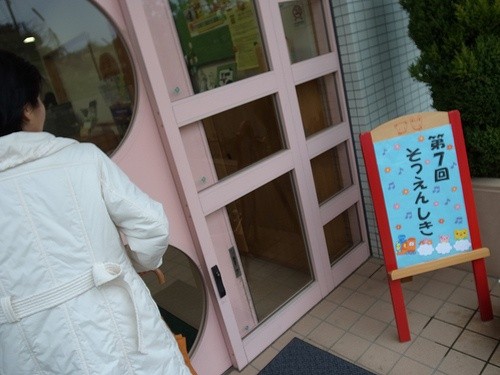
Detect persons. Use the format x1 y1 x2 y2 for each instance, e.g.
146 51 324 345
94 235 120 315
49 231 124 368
1 49 190 374
